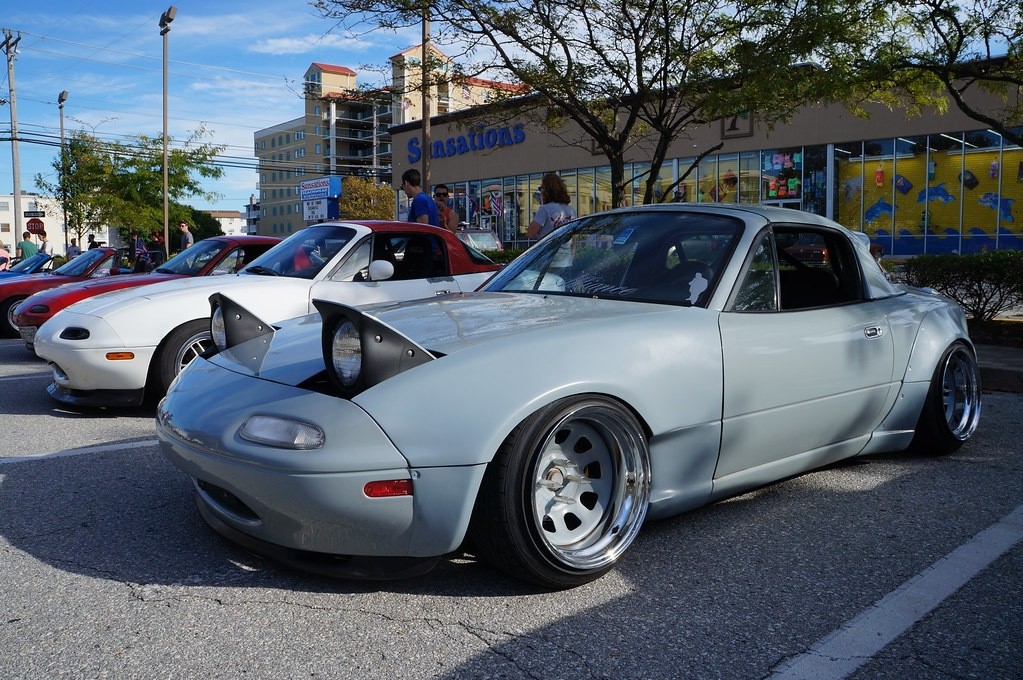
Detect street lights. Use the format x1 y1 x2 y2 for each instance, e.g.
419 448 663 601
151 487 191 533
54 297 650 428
54 90 68 254
157 5 177 258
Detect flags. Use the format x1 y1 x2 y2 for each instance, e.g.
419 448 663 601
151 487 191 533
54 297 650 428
491 193 502 217
469 196 478 218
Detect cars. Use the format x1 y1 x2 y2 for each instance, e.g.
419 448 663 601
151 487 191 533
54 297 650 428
391 218 505 265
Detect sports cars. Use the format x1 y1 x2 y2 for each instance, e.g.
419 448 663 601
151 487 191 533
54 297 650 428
13 234 308 352
33 217 568 413
1 244 131 338
157 198 984 590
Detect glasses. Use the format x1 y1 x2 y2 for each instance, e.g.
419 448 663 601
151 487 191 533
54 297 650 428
179 224 186 227
400 181 405 190
435 191 448 197
538 186 544 190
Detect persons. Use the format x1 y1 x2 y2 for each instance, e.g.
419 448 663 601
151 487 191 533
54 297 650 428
177 219 193 252
38 230 53 256
16 232 38 261
66 238 81 262
526 174 575 241
402 169 439 226
88 234 98 250
151 231 167 265
0 240 10 272
433 184 459 230
